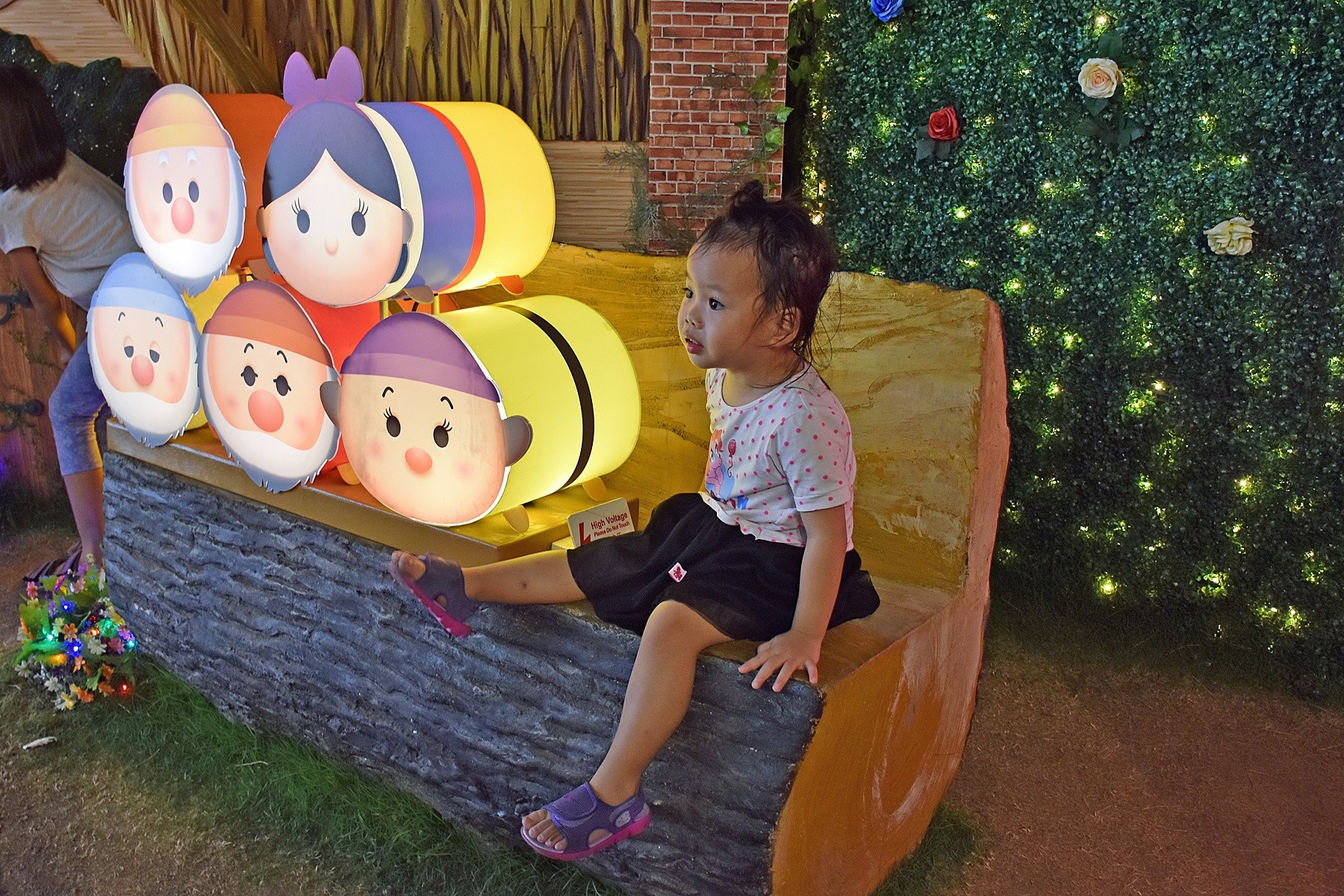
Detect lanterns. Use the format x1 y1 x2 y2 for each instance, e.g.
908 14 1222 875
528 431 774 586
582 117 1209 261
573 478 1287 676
87 46 644 528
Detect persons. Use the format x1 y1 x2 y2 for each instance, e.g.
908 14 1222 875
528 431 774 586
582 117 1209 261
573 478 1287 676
389 180 881 860
0 61 145 598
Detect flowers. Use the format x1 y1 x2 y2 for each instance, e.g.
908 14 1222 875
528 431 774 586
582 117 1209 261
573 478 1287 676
915 103 967 167
1072 30 1145 143
1203 213 1255 259
12 553 138 713
868 0 903 23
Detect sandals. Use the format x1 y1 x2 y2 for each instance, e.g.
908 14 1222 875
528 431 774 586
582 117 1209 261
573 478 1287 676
388 552 483 639
522 783 651 861
24 543 108 600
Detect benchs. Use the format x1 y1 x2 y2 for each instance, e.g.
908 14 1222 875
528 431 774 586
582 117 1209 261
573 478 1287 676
101 236 1012 896
